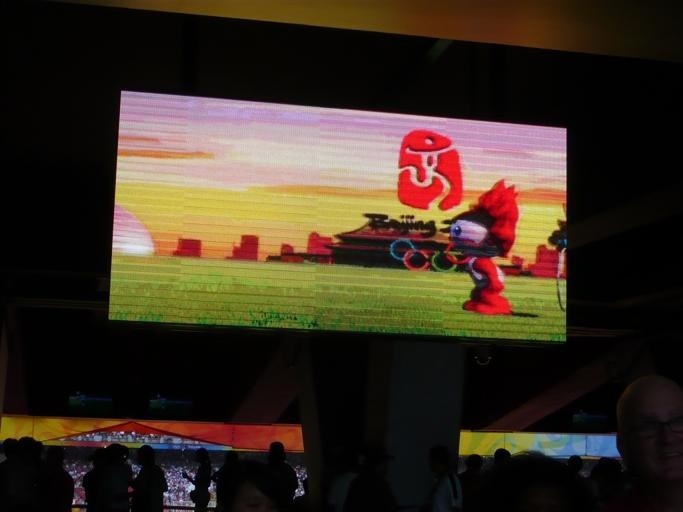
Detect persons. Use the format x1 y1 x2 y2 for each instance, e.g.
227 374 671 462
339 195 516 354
1 425 683 511
589 371 682 511
